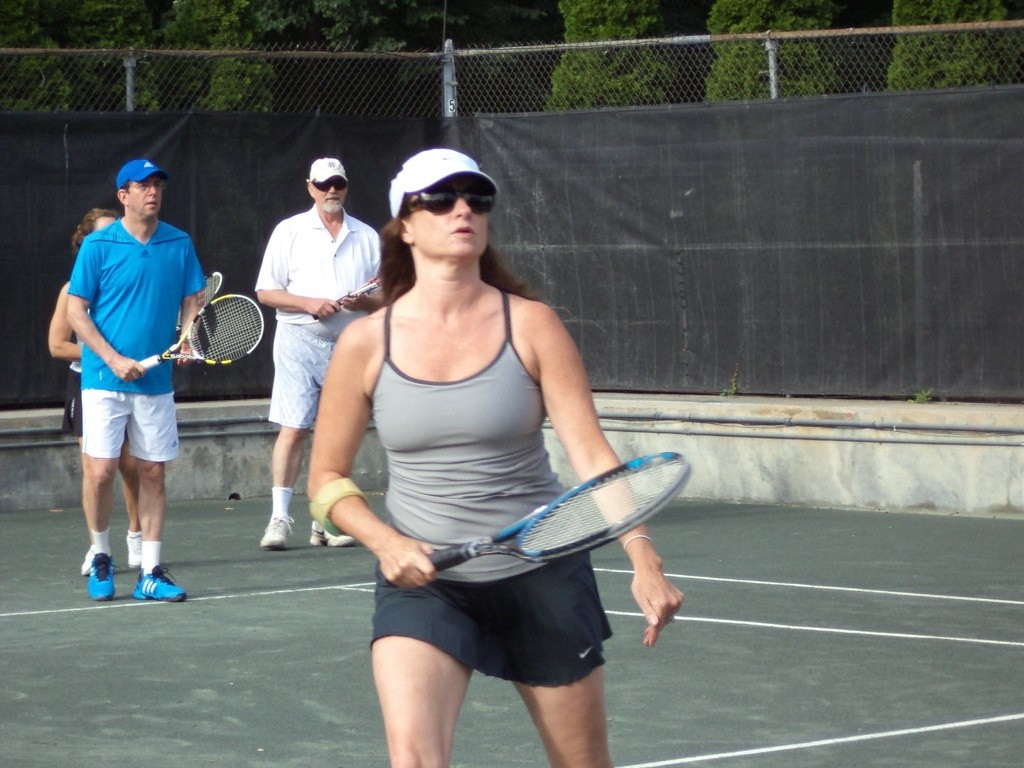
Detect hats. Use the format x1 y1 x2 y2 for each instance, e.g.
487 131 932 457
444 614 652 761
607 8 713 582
389 149 499 219
115 159 169 191
306 158 348 183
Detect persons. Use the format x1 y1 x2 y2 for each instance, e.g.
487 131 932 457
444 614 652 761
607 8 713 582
47 208 142 574
65 160 207 604
307 149 686 768
254 157 384 547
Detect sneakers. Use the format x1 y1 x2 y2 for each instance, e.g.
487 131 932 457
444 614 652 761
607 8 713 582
87 554 114 601
260 516 294 546
81 545 100 575
126 535 142 568
134 566 186 601
310 521 355 547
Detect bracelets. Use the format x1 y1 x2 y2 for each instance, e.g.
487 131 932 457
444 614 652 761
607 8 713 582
623 535 652 551
308 478 369 535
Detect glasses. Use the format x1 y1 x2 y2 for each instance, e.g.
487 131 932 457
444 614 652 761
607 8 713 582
312 180 347 192
404 179 496 213
129 180 167 190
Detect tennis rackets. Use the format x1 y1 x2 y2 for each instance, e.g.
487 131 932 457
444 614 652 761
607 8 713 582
176 270 224 332
386 450 692 589
313 274 385 322
138 293 265 370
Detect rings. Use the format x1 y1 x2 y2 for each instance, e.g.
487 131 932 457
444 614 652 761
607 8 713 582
666 616 675 621
